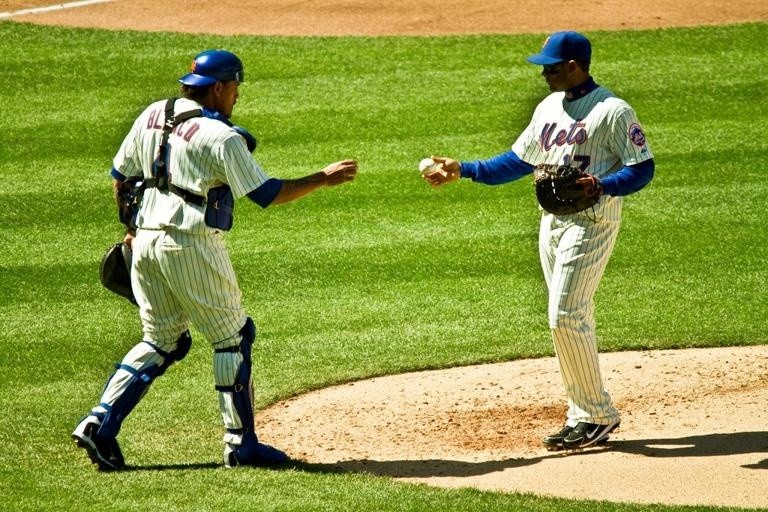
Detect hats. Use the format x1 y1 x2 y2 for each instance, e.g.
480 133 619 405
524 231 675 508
527 31 592 65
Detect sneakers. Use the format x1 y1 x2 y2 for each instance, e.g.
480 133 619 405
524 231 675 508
563 419 624 450
71 416 126 470
224 443 289 467
543 426 574 452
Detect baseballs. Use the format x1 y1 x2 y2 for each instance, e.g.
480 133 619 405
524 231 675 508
419 158 436 175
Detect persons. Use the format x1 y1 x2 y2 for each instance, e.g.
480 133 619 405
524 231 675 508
420 29 658 450
67 50 358 472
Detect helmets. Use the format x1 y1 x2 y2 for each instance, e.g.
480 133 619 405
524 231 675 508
178 49 245 88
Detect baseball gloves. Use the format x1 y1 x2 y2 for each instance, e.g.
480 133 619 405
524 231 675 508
533 164 603 225
100 243 138 308
